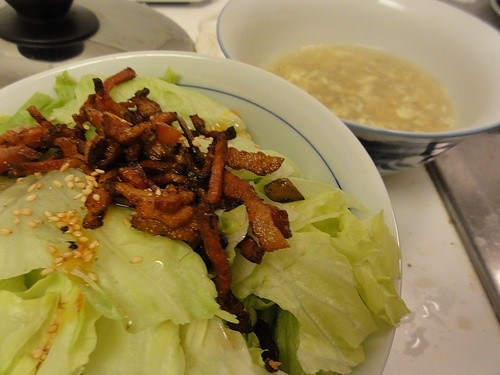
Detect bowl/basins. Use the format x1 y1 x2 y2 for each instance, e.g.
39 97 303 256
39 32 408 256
0 50 401 375
216 0 500 175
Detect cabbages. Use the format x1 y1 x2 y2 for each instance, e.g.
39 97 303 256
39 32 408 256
0 68 413 375
